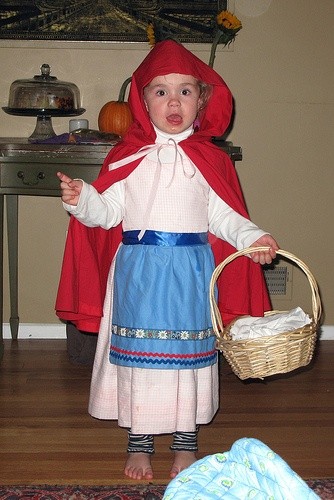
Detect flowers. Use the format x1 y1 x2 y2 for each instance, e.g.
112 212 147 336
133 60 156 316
146 10 243 68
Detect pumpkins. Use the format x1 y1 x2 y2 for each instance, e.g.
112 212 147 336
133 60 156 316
98 77 134 137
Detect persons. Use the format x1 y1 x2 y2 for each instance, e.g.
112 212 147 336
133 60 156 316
55 40 280 480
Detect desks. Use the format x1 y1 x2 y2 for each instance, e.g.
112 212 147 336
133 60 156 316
0 137 242 342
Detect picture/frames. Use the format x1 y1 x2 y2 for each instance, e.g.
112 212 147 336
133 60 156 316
0 0 235 52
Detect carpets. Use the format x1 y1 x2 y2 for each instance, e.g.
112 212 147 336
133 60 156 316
0 476 334 500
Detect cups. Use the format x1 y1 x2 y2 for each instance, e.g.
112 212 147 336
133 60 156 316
69 119 88 132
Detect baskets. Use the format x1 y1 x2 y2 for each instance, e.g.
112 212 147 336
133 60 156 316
208 247 322 381
